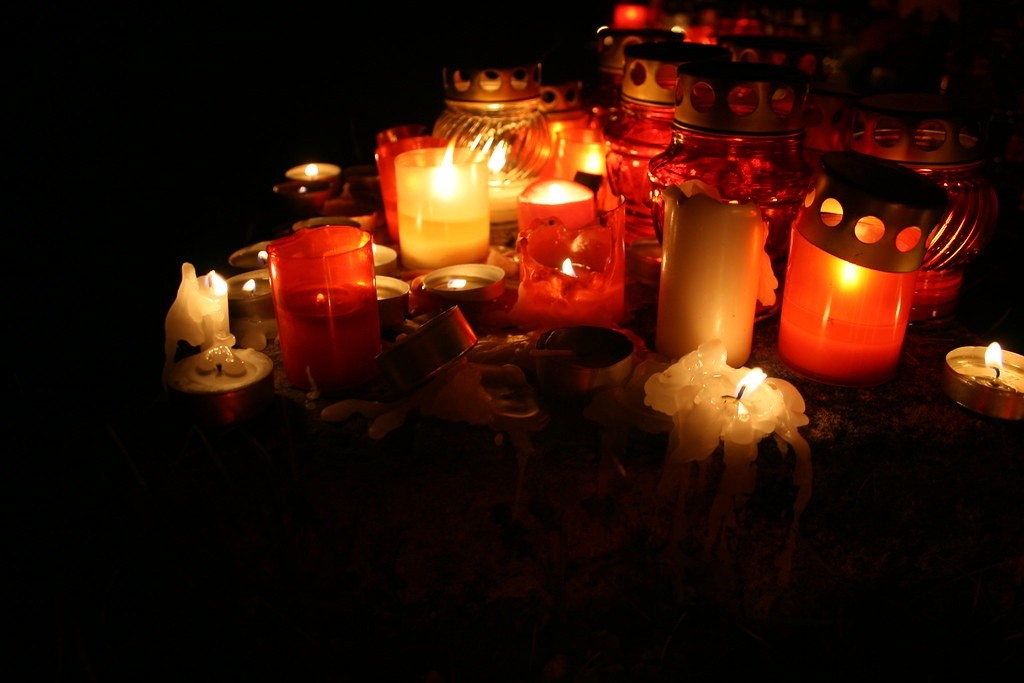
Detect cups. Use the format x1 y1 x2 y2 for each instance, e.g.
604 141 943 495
394 147 490 271
267 225 381 398
654 186 764 367
516 179 626 332
377 135 448 241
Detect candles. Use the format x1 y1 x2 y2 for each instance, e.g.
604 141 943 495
167 139 1024 467
